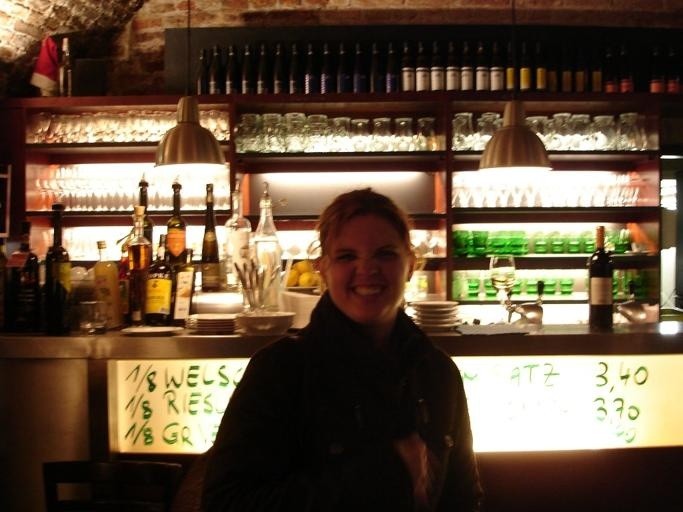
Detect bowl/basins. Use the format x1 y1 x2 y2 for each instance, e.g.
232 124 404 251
240 312 294 334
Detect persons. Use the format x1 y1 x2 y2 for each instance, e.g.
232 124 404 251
194 187 484 511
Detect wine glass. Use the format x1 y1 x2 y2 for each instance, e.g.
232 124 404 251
489 253 514 314
30 110 230 146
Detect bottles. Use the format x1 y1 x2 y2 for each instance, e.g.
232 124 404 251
0 180 70 336
58 35 74 95
94 242 124 330
128 182 281 323
191 38 681 93
589 225 613 330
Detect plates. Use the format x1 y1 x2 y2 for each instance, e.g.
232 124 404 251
186 314 242 334
413 298 461 333
285 285 319 292
120 327 184 335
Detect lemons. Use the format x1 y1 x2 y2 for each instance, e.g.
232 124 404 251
287 260 325 287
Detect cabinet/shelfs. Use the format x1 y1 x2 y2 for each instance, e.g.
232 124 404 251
7 92 659 322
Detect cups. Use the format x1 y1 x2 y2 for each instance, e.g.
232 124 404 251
237 109 439 152
449 111 645 151
76 301 104 331
455 185 645 299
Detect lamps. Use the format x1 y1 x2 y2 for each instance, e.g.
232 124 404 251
153 3 227 181
477 3 555 182
